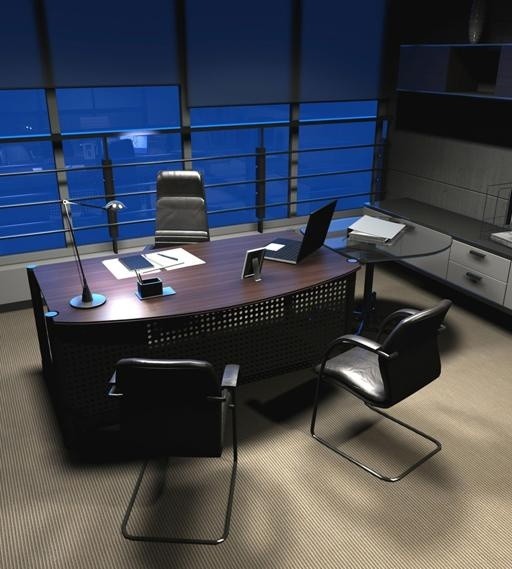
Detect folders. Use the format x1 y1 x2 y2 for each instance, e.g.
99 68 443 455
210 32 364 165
347 214 406 247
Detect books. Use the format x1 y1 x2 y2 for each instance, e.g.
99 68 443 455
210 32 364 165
490 231 512 248
118 255 155 273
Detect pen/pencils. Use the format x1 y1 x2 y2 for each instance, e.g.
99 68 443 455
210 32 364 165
157 254 178 261
135 269 143 283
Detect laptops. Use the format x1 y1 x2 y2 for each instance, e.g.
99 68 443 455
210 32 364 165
262 199 337 265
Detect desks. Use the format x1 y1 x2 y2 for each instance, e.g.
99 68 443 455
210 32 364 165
301 212 453 331
26 228 360 423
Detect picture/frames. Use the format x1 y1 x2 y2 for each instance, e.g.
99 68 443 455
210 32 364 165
241 247 266 279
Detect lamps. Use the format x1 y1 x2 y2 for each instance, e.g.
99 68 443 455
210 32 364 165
62 197 128 308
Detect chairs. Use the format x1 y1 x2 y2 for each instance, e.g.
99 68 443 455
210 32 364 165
138 171 210 254
107 355 240 546
310 300 451 482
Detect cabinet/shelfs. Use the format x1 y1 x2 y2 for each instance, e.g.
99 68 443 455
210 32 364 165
360 205 512 332
